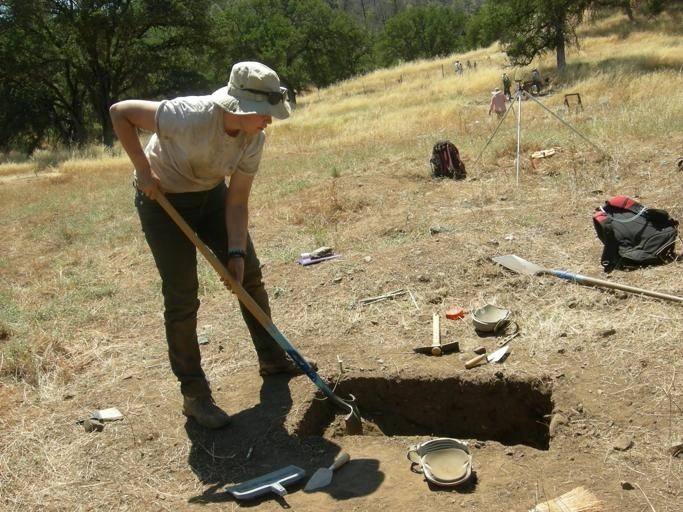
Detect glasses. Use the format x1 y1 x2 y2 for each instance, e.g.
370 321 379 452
239 86 287 103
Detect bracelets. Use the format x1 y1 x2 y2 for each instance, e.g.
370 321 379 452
227 247 247 259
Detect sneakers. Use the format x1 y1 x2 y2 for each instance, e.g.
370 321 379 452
181 394 230 431
258 355 319 379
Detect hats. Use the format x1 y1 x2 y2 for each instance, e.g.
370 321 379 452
489 88 500 96
208 60 294 120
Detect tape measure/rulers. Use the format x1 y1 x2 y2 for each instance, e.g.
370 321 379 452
446 303 463 320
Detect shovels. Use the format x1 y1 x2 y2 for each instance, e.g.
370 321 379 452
226 465 305 500
135 175 362 434
491 254 683 303
303 452 350 490
465 345 509 369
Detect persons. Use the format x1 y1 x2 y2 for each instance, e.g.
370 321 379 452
488 88 508 119
502 73 511 102
454 63 463 78
454 60 459 78
110 59 316 429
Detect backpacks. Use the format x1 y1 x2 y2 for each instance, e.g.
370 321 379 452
430 138 467 181
590 194 678 275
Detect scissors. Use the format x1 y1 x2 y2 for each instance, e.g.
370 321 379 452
362 288 406 306
